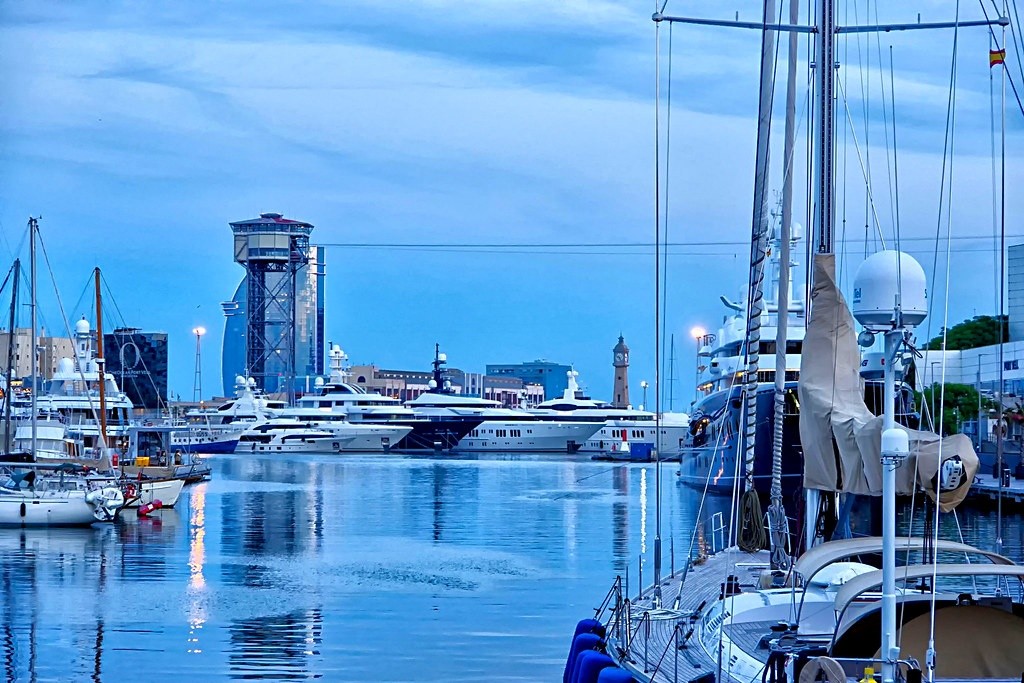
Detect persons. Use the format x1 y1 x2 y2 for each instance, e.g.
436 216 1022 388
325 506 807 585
160 451 166 467
174 449 182 465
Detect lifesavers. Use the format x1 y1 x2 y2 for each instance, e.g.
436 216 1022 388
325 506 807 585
798 655 847 683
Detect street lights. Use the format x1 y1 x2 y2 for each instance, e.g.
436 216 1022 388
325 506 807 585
690 326 707 402
191 327 206 403
641 381 649 410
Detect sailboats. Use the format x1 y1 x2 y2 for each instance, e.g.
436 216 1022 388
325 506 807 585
0 1 1024 680
